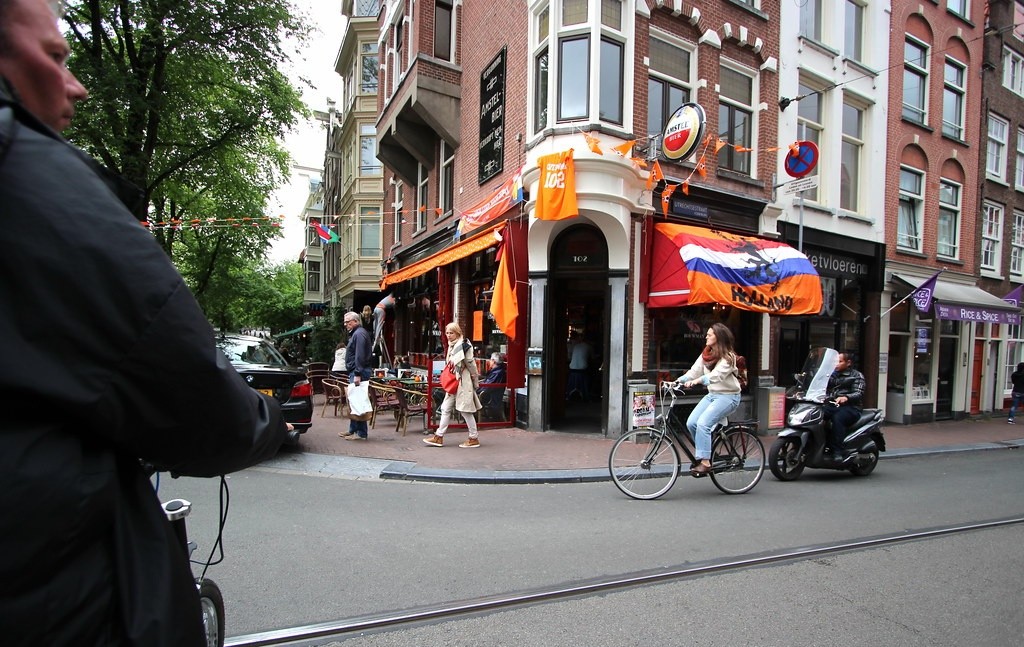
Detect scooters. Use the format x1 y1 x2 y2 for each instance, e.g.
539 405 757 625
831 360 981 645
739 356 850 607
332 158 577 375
768 346 887 482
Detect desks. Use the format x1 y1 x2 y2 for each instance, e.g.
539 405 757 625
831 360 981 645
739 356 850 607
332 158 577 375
401 380 439 422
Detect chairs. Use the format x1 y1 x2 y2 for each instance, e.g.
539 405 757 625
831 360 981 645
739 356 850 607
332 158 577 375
477 383 509 429
306 363 436 438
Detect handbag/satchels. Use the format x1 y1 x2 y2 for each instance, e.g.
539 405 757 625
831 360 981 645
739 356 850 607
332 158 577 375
440 360 458 395
343 380 373 422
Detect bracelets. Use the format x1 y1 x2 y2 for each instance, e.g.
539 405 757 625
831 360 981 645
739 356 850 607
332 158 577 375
843 395 849 402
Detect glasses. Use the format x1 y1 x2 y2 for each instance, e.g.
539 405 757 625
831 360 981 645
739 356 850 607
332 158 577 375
342 319 355 323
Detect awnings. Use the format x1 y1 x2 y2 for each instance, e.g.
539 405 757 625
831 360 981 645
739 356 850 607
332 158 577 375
644 223 824 318
379 218 510 290
891 274 1022 327
273 323 315 341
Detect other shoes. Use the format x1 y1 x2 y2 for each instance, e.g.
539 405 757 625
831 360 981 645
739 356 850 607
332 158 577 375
1007 418 1016 425
690 463 711 474
833 451 844 463
344 432 365 441
338 431 353 437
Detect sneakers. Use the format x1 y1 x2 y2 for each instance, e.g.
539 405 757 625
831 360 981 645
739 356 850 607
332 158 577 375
422 434 444 447
458 437 480 448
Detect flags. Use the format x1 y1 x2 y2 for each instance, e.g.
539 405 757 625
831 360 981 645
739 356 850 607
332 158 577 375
1001 284 1023 308
311 220 342 244
490 223 518 341
910 270 943 313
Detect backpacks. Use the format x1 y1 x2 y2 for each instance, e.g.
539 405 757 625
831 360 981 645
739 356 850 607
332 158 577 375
732 354 748 386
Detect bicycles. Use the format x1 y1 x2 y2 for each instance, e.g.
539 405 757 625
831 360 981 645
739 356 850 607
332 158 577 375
608 380 767 500
128 425 304 647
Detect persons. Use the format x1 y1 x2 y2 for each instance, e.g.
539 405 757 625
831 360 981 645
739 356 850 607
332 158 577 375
361 305 375 338
338 311 373 441
396 355 411 369
664 324 742 474
423 321 486 448
474 353 506 406
1007 362 1024 425
332 343 348 404
558 330 601 407
801 352 866 460
1 1 295 647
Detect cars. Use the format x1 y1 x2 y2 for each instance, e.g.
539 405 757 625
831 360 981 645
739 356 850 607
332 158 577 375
210 330 315 437
